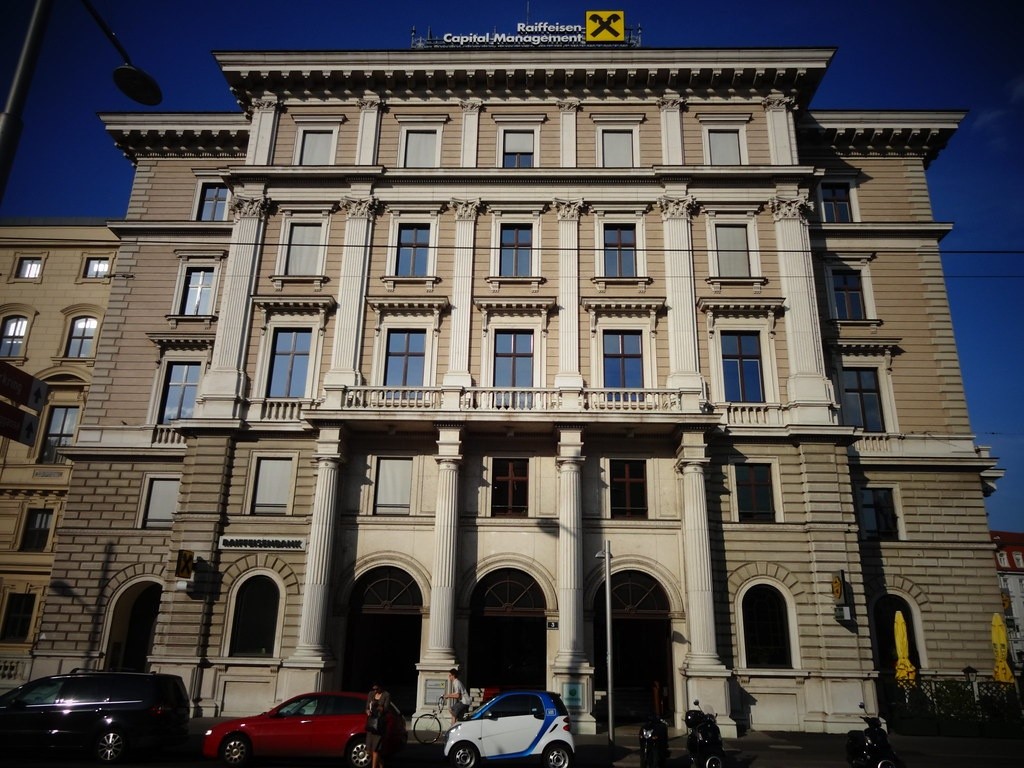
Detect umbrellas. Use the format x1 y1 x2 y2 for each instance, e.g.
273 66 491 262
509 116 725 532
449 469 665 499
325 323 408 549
990 613 1015 722
894 610 916 708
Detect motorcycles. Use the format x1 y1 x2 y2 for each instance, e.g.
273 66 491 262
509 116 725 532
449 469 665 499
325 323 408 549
638 719 669 768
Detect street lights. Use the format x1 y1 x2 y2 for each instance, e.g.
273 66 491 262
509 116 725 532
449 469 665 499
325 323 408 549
1 1 166 209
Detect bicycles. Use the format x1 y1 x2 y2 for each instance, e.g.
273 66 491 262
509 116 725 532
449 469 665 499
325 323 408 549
412 694 471 744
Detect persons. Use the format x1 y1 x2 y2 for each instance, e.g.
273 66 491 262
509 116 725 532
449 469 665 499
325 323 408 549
365 681 391 768
443 668 471 721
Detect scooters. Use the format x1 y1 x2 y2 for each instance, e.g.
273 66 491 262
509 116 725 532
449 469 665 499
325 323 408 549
844 702 899 768
684 700 727 768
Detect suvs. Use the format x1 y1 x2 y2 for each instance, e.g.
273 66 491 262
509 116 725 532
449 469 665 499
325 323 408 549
0 668 191 768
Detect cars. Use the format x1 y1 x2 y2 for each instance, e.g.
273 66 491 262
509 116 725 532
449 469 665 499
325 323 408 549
203 691 407 768
443 691 575 768
